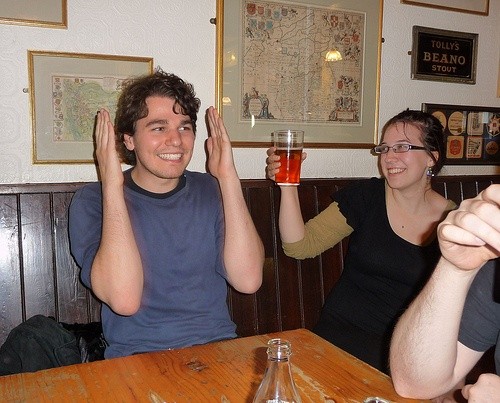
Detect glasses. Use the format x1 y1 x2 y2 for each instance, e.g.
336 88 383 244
374 143 434 154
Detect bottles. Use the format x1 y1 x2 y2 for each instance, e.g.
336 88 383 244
254 338 301 403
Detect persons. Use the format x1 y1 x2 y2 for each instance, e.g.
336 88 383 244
67 69 265 361
267 107 460 374
388 184 500 403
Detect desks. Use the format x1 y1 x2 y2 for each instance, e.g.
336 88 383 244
0 329 430 403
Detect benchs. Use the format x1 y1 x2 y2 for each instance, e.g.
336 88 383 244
0 175 500 384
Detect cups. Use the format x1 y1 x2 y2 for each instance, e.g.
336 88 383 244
273 129 305 186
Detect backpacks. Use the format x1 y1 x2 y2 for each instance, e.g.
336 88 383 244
0 314 88 378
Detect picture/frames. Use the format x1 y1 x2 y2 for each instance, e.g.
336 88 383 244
400 0 490 16
0 0 67 29
411 25 478 84
421 103 500 165
27 49 153 165
216 0 384 148
